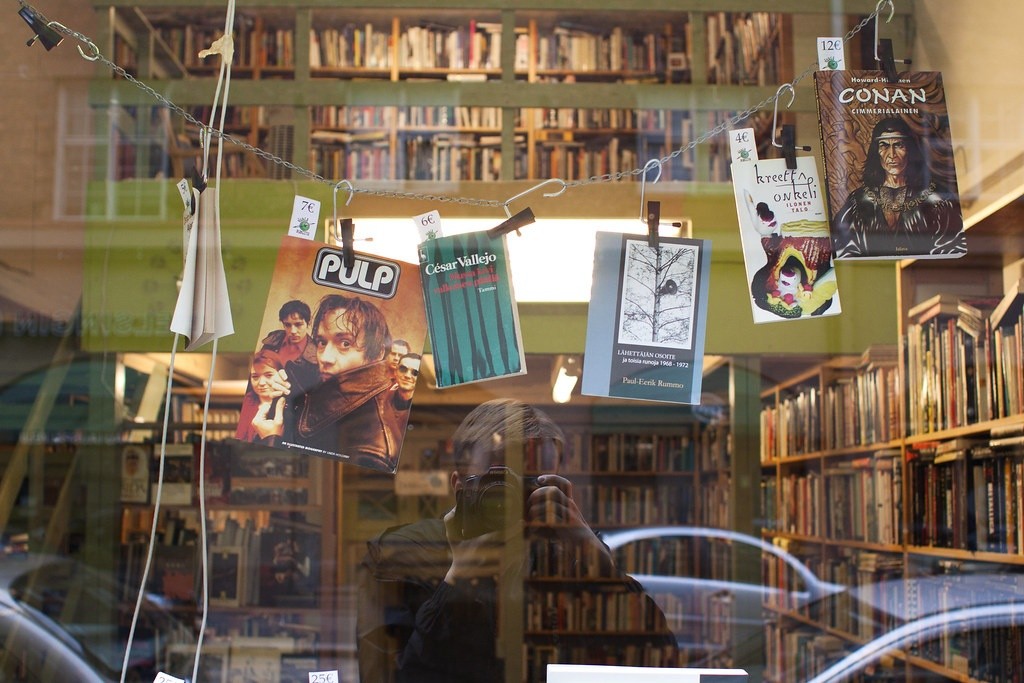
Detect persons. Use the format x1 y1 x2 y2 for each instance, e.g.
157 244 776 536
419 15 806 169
347 396 682 683
235 291 423 473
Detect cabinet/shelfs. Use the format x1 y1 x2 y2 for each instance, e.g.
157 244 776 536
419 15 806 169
86 0 795 188
2 185 1024 677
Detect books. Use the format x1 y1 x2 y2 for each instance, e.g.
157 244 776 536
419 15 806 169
519 420 737 683
167 182 237 353
724 156 843 325
230 234 429 473
580 231 714 407
119 391 322 683
416 229 529 391
109 8 796 188
812 69 969 261
758 287 1024 682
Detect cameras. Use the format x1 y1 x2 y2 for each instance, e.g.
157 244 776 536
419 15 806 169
456 465 559 543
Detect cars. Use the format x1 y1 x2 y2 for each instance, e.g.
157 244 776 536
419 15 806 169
684 569 1024 683
600 530 854 657
0 547 223 683
